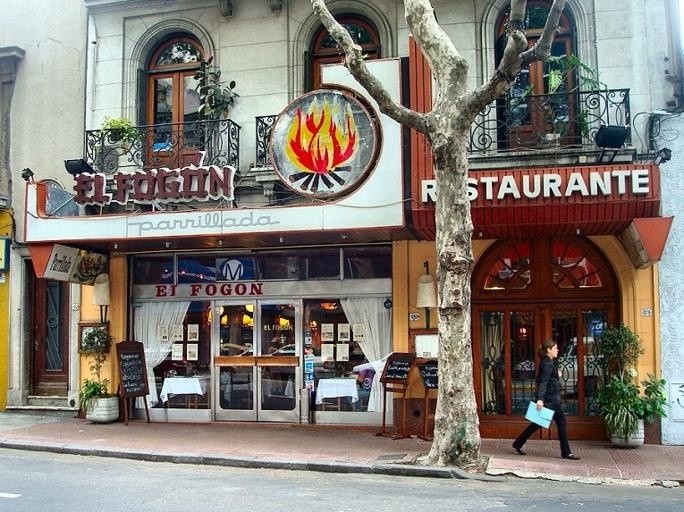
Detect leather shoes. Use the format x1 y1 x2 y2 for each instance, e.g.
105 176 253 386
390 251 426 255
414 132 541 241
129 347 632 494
512 441 528 456
564 452 581 460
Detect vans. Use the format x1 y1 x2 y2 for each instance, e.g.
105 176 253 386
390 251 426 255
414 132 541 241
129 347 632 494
556 336 610 397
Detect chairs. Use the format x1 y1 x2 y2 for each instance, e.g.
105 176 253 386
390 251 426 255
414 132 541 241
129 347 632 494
228 367 252 410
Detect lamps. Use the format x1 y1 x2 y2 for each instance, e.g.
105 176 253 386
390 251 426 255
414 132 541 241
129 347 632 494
63 157 96 178
594 124 629 164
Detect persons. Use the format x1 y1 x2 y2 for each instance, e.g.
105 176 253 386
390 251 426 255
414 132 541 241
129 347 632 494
511 339 580 460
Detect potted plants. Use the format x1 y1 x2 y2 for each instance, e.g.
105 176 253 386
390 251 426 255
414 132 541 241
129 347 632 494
97 113 144 148
78 319 122 425
589 321 671 450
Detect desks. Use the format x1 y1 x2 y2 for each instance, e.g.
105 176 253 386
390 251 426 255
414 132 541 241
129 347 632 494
316 376 359 410
222 372 255 399
163 374 209 409
260 378 297 409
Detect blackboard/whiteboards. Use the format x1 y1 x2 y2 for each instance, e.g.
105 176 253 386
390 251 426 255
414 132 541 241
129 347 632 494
116 341 150 398
380 352 438 389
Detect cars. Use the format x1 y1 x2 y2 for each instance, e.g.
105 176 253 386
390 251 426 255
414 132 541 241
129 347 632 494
219 343 394 392
153 356 192 379
